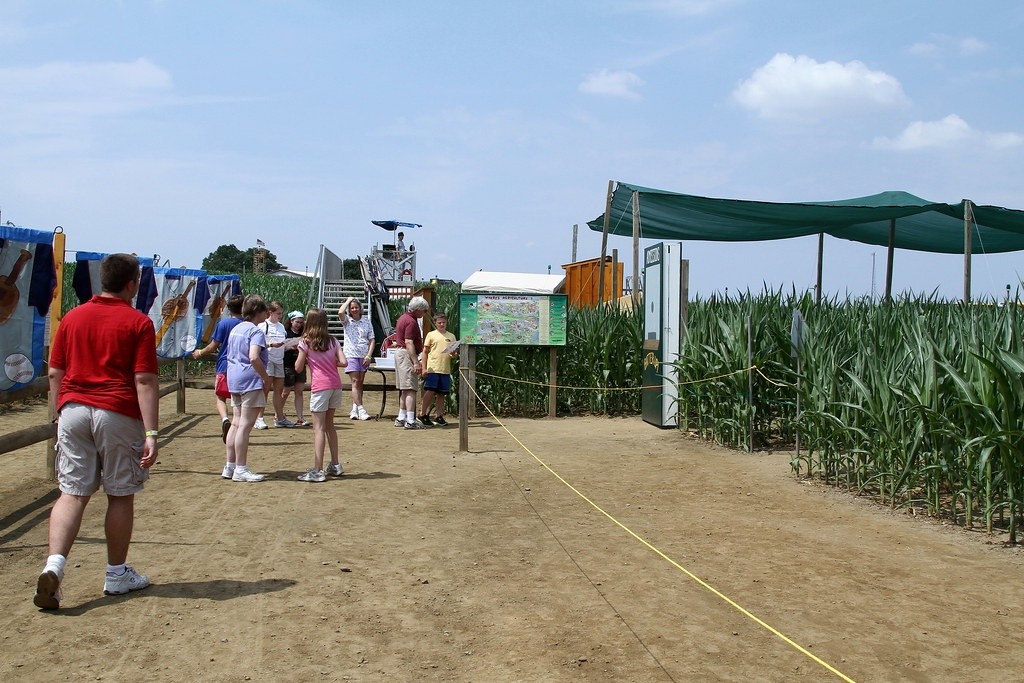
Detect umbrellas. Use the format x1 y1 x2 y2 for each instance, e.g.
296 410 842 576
371 220 422 281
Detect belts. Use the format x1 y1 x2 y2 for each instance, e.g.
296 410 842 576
398 346 406 349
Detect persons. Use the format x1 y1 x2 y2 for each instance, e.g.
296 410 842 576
294 309 348 482
338 296 375 421
273 310 310 426
416 312 458 426
190 294 245 444
254 301 294 430
394 296 430 431
34 252 160 610
221 294 270 483
395 231 407 282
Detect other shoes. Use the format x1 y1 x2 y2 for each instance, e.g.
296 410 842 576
349 409 358 420
325 462 345 476
221 466 235 478
254 417 268 429
275 418 294 427
394 417 406 427
416 413 434 427
232 469 264 482
221 419 231 444
358 409 371 420
298 467 326 481
404 421 426 430
434 415 448 426
296 416 309 426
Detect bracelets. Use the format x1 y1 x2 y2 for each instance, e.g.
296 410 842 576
146 430 159 437
198 350 203 357
269 343 273 347
366 356 371 360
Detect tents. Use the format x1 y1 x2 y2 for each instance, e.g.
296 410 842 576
586 181 1024 321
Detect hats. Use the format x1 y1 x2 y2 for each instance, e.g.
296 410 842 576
288 310 304 320
398 232 404 236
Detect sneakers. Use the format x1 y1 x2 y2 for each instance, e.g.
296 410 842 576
32 563 64 610
103 566 149 594
